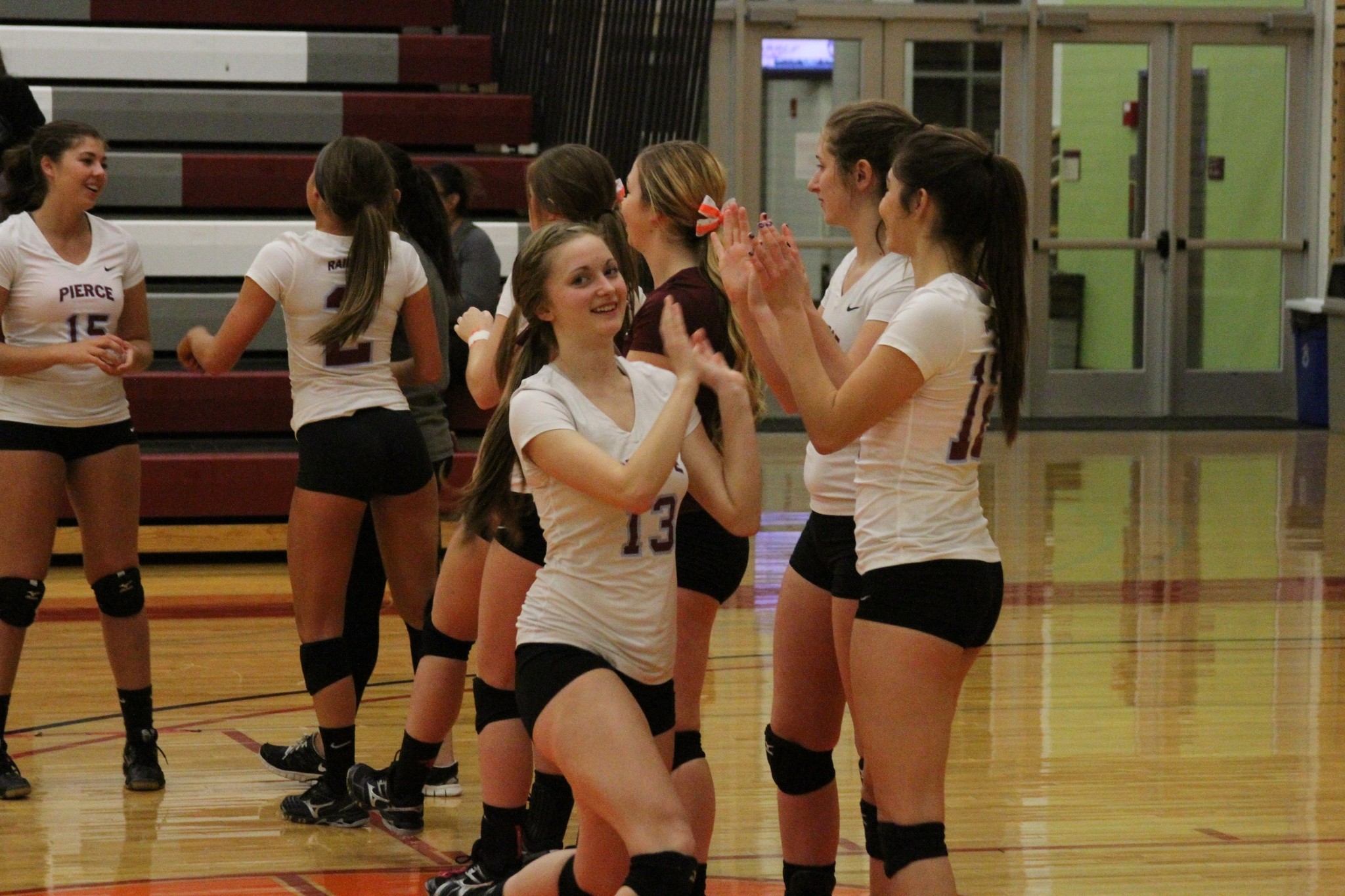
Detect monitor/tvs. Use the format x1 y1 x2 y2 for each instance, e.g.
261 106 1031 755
759 38 835 76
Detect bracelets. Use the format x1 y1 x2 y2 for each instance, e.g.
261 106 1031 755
468 329 491 347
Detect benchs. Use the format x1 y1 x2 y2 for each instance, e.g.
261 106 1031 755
0 1 544 570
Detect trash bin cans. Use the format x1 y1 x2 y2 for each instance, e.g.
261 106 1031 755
1285 300 1328 427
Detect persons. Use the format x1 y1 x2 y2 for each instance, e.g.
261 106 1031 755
0 118 170 798
178 135 647 835
432 224 761 896
747 128 1026 895
619 142 752 896
708 100 985 896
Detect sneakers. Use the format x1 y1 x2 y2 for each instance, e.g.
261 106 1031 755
471 831 563 867
346 750 426 835
426 854 523 896
280 776 372 826
0 741 32 799
121 728 168 790
422 760 463 798
260 732 327 785
